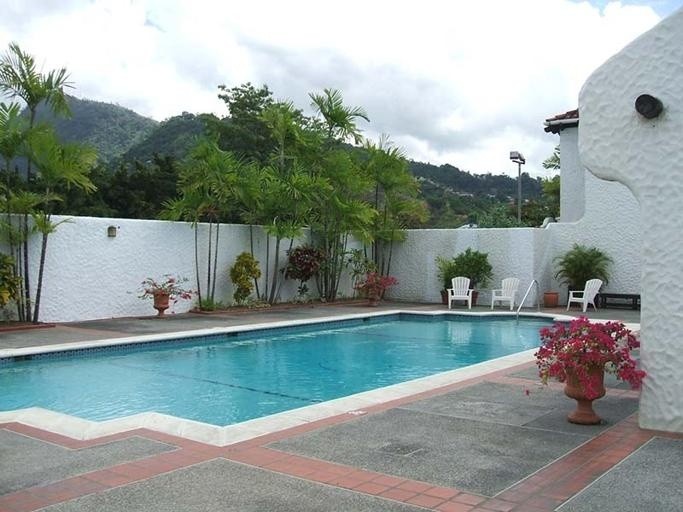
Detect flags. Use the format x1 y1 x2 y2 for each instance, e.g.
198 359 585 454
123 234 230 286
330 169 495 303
562 353 609 424
543 292 558 308
152 292 169 318
367 297 378 306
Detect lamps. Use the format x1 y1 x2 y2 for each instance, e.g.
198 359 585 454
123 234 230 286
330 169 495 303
446 276 474 310
491 277 520 310
566 278 603 313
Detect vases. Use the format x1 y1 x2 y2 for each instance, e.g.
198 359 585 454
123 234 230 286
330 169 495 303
352 270 400 297
125 273 200 307
524 313 648 402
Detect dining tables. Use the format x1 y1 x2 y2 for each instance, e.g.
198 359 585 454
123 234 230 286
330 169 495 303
552 242 614 308
433 247 494 306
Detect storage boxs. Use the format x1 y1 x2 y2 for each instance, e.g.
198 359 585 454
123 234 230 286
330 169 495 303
601 292 640 310
